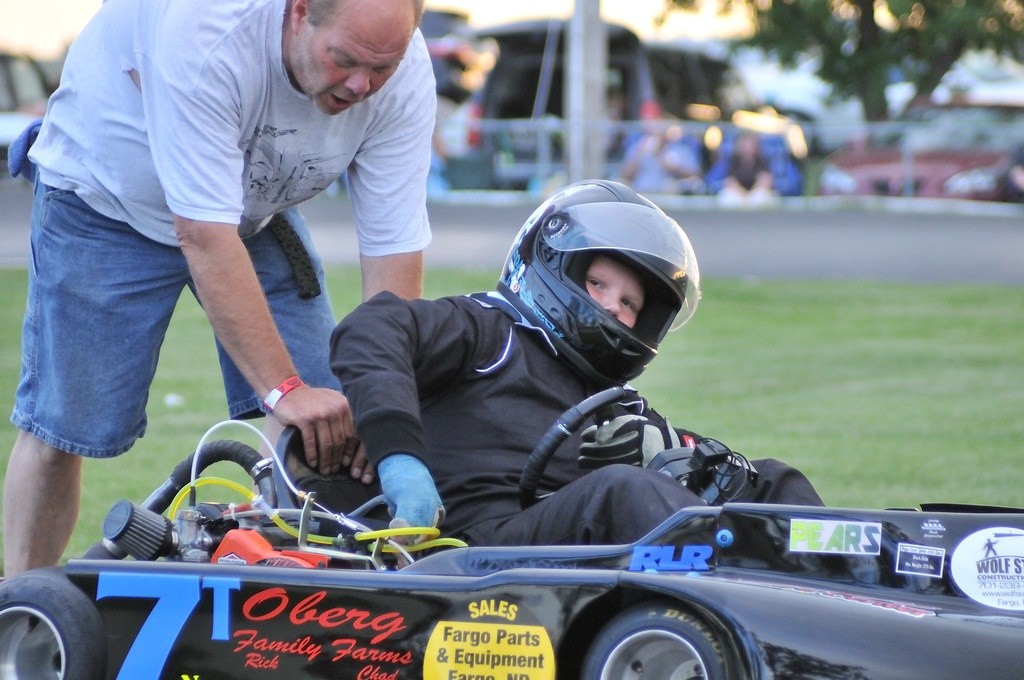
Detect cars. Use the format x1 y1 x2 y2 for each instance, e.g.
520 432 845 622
817 67 1024 205
0 6 841 198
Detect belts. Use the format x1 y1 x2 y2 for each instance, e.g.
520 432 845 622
268 213 321 299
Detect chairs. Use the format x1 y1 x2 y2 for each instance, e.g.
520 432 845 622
276 424 392 536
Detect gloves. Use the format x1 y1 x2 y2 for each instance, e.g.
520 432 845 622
578 383 705 487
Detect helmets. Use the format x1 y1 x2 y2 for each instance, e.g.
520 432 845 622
496 179 702 390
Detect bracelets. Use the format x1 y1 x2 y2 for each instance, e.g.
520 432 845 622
262 376 304 411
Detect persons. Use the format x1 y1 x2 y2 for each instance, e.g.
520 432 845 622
722 130 773 194
622 128 700 189
329 178 825 558
3 0 437 577
